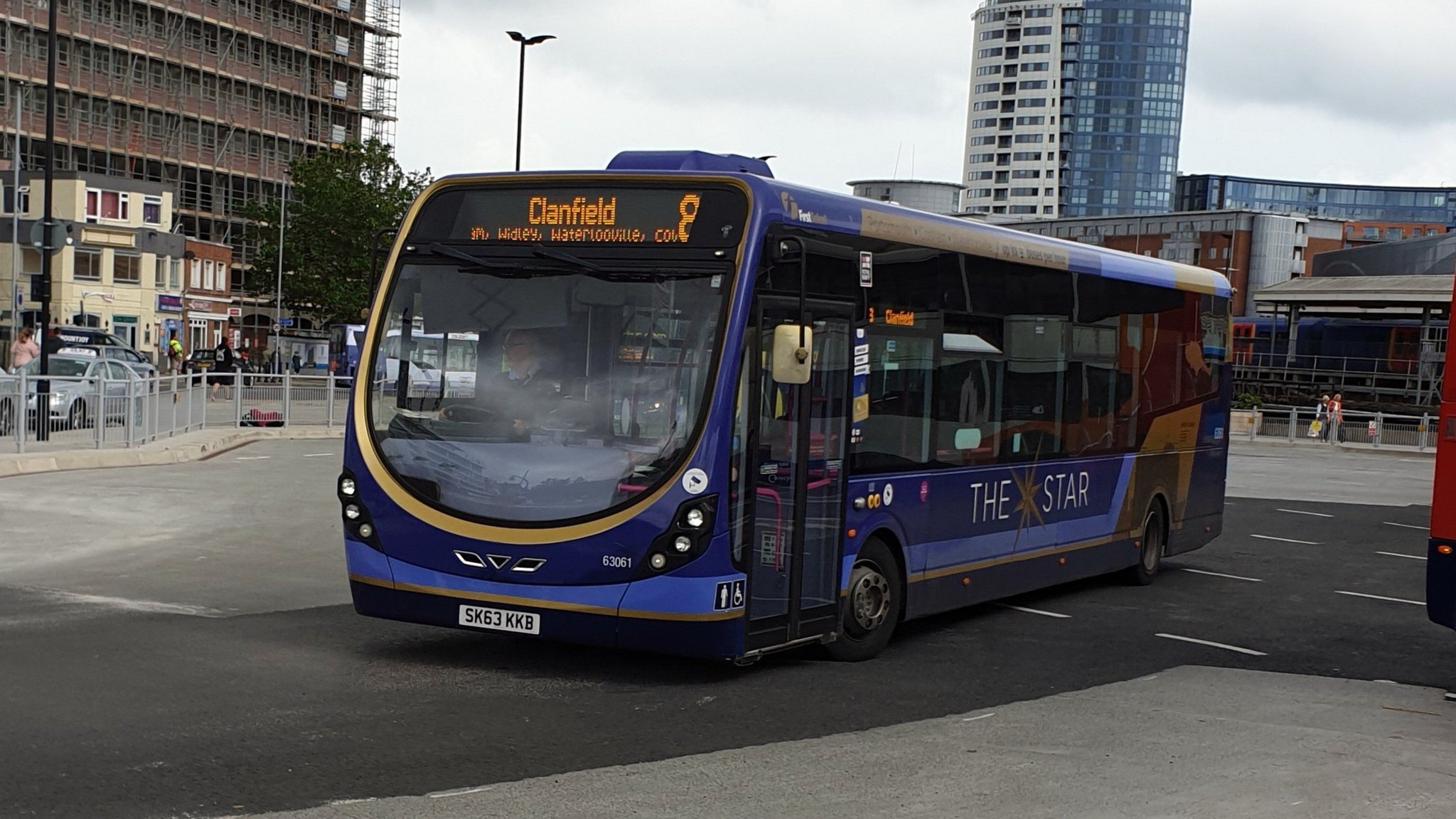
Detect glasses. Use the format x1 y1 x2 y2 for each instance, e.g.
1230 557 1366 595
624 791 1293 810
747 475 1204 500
501 343 526 351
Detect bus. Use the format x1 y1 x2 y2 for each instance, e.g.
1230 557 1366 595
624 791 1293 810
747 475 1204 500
328 323 404 389
334 150 1234 667
381 328 480 409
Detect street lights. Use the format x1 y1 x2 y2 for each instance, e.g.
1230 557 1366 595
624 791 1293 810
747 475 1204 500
505 31 558 171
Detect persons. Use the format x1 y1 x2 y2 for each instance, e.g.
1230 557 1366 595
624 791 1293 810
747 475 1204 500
292 351 301 379
261 346 281 373
439 329 567 443
168 337 184 375
7 327 66 375
1316 395 1329 443
210 337 233 402
1327 394 1343 443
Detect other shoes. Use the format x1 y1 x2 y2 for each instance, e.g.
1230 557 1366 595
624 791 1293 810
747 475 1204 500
271 380 275 383
176 368 181 375
225 398 234 402
210 394 215 401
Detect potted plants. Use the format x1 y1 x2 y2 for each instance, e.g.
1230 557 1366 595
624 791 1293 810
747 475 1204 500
1229 393 1263 435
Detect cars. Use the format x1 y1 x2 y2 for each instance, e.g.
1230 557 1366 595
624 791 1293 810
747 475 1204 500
0 363 28 436
181 348 255 387
12 353 146 432
67 344 156 393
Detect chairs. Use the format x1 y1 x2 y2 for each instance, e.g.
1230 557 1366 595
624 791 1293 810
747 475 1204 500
771 352 1198 469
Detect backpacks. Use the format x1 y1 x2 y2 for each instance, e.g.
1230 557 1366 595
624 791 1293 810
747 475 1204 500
168 343 180 357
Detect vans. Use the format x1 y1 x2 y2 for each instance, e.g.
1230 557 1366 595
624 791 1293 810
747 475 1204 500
33 323 151 364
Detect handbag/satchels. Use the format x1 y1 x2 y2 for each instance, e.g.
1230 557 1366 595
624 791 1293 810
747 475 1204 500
1306 420 1321 438
